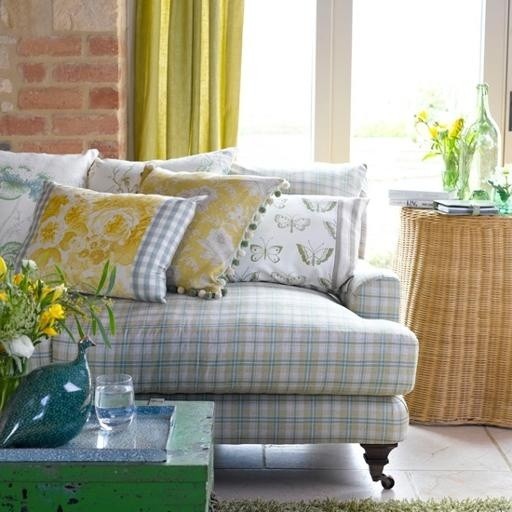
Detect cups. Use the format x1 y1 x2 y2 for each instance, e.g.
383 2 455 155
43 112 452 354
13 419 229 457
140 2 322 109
94 374 134 432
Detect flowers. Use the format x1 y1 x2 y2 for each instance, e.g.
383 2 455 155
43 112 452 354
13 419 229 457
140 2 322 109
410 110 466 168
2 245 117 413
486 170 512 203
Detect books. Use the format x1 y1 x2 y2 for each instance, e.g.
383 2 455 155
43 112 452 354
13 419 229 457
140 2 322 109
391 199 436 209
435 198 498 216
389 189 450 201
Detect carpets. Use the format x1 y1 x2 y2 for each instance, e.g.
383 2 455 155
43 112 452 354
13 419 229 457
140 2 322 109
207 496 512 512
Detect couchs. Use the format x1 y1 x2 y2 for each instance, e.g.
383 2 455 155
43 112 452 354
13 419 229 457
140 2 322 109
28 191 420 492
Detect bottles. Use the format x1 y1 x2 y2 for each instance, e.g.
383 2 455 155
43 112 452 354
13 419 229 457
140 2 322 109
461 79 502 204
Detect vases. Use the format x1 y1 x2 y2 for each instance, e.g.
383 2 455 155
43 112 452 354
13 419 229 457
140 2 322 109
493 194 512 215
441 169 459 193
